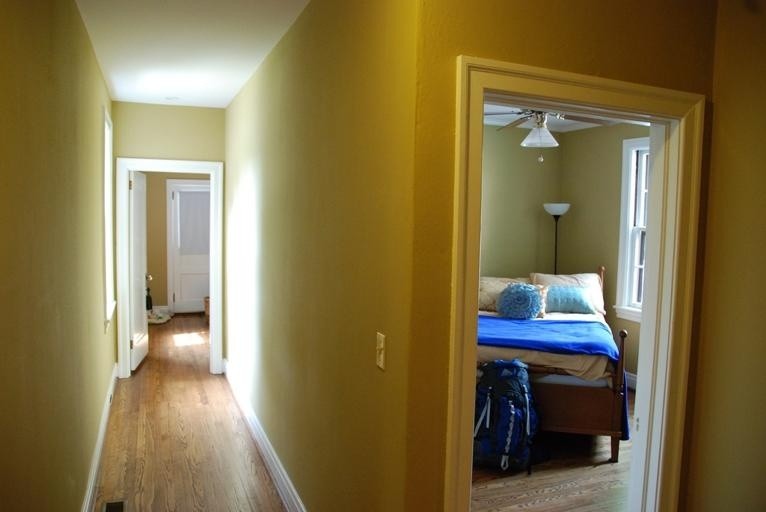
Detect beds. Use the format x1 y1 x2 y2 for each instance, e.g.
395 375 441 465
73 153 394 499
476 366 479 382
474 266 627 463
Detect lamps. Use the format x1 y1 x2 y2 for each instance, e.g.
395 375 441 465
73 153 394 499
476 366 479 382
542 203 572 275
520 112 560 163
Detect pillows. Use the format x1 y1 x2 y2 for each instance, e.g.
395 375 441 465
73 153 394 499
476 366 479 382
546 285 597 315
496 283 543 319
479 277 530 311
529 272 606 314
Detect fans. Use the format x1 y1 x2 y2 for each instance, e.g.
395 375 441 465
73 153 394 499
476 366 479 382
483 109 615 132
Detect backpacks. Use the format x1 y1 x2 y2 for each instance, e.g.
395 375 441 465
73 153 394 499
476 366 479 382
473 358 537 475
